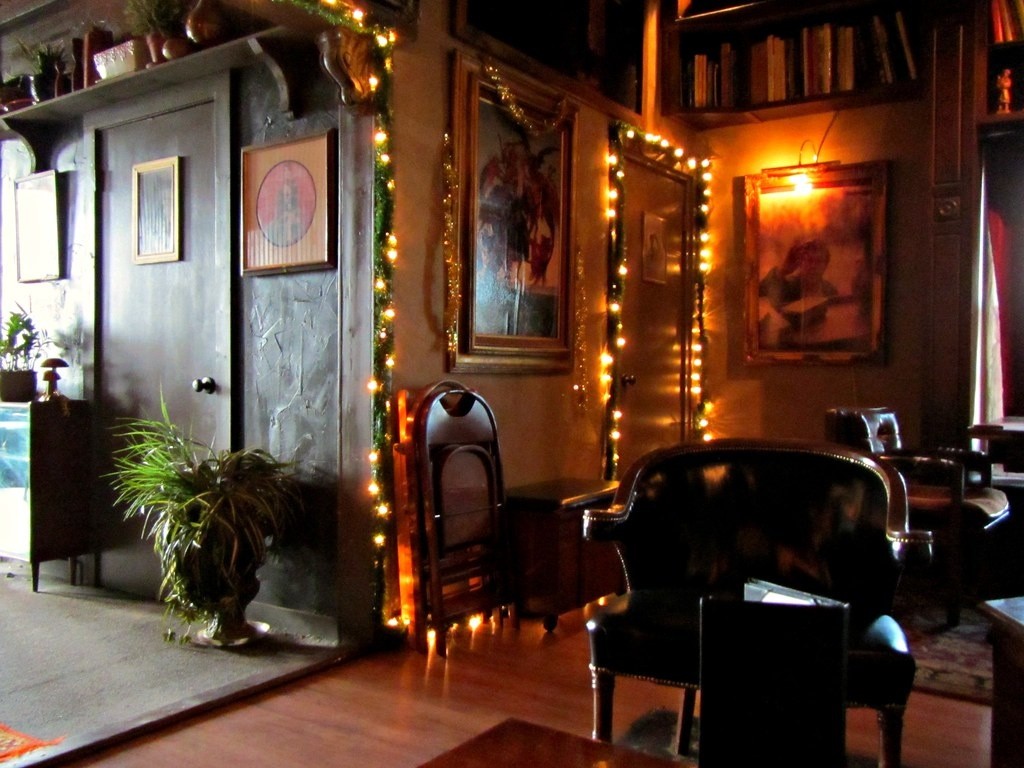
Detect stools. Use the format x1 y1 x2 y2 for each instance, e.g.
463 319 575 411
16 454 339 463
500 476 632 633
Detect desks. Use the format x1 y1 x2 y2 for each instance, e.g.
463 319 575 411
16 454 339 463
978 597 1024 768
414 717 694 768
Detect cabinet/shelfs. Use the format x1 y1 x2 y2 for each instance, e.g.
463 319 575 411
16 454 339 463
0 399 117 592
656 0 927 132
973 0 1024 132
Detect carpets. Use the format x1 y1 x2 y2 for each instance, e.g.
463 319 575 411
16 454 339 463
0 723 66 763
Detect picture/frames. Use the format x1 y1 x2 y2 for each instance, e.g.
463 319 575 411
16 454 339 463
131 155 182 264
445 46 579 376
641 210 668 286
12 169 63 284
741 158 893 366
238 126 338 278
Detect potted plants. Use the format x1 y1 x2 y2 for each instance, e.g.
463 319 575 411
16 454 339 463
0 297 53 402
98 379 304 649
0 0 229 116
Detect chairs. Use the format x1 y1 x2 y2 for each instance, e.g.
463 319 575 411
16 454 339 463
392 378 511 659
581 435 933 768
826 405 1011 628
